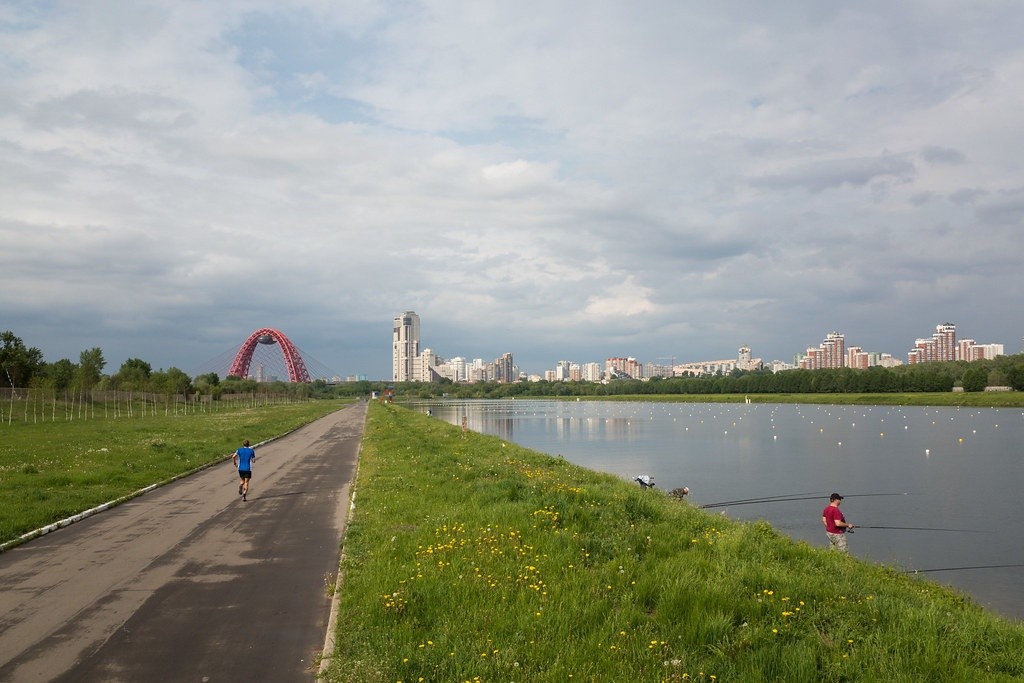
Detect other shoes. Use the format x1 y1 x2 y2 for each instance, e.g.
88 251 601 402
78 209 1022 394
239 485 243 495
242 497 247 501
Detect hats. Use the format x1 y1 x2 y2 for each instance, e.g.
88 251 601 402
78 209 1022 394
830 493 844 499
685 487 689 496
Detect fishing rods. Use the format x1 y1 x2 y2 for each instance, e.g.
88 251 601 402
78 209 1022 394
901 563 1024 574
847 525 995 534
696 492 909 509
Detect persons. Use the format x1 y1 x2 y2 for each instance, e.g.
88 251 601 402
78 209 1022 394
822 492 855 555
668 486 689 499
233 439 256 502
388 389 394 404
